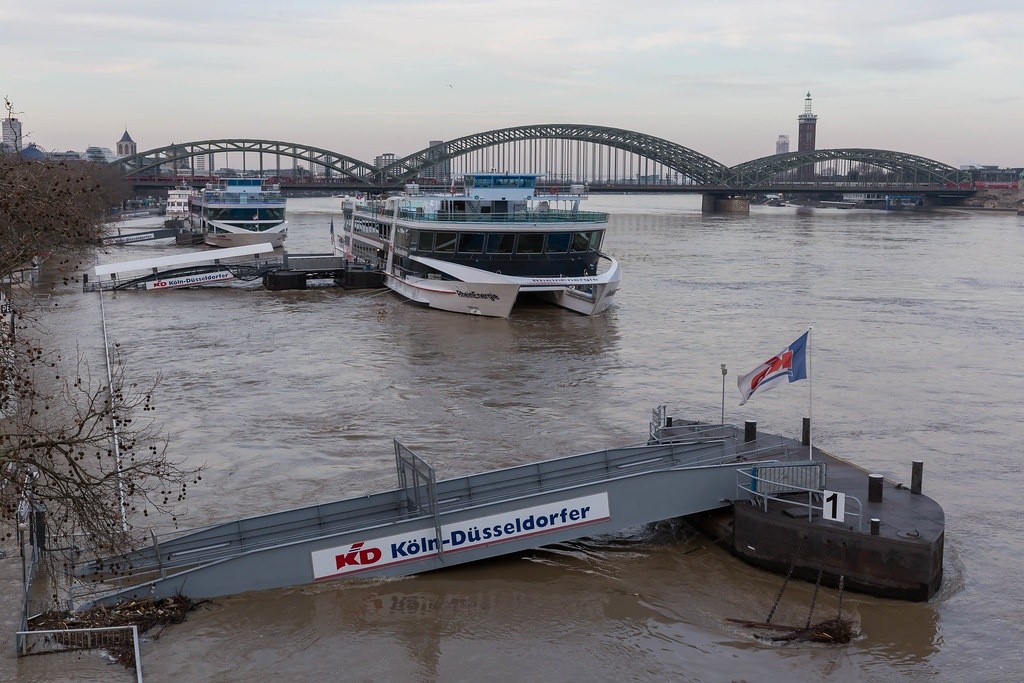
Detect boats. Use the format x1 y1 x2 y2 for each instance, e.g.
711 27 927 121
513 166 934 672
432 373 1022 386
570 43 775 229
330 169 622 318
166 177 288 247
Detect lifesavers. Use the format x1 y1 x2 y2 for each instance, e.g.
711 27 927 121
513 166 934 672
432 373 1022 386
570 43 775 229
380 208 385 214
357 195 361 199
551 186 557 194
449 186 457 193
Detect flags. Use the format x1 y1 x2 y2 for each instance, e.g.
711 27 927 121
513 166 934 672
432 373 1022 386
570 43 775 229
737 330 810 407
